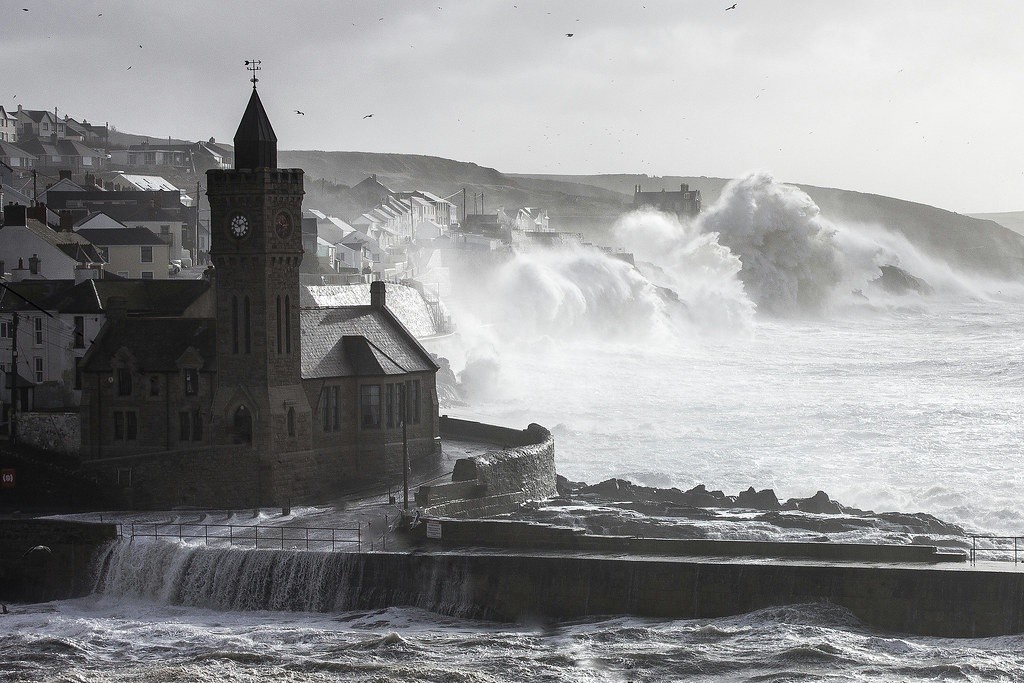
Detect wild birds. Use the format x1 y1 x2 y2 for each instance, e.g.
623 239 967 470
9 4 751 152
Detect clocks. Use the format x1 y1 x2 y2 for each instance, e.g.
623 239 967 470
275 213 291 237
227 211 250 239
